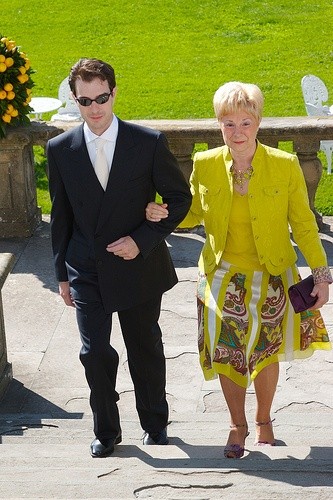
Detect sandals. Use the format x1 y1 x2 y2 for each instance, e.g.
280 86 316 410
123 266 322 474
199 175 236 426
224 424 249 458
254 420 275 447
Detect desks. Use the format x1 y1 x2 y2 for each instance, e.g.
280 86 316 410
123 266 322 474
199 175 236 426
26 97 62 120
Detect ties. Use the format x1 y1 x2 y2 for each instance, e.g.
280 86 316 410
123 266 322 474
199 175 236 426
94 138 109 191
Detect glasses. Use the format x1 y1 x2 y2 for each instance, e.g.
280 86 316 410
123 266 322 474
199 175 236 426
75 92 113 106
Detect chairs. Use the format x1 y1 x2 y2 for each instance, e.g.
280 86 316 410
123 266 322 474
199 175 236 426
51 76 82 122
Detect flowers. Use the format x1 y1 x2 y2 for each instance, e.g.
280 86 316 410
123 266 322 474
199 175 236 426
0 33 36 138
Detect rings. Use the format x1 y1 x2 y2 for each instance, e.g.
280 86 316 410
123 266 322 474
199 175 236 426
150 213 153 218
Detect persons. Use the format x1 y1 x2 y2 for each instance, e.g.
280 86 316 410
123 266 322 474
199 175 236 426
146 81 333 457
47 57 193 458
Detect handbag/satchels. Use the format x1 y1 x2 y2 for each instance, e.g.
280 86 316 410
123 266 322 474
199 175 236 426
288 275 317 313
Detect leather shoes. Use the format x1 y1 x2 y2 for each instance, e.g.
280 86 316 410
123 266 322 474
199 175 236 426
143 421 168 445
91 430 122 458
